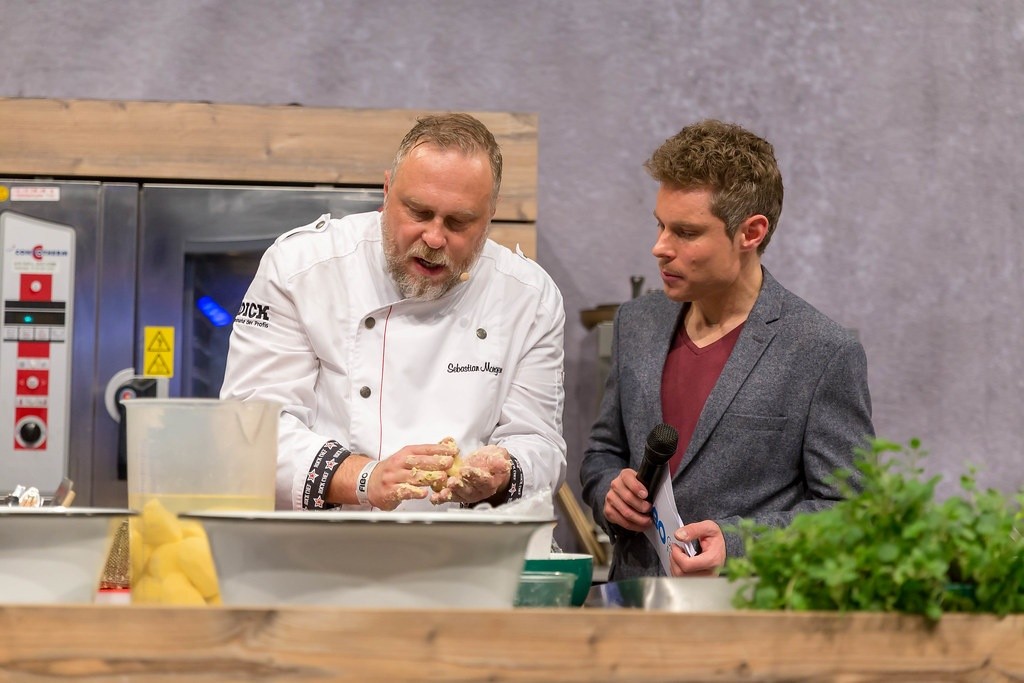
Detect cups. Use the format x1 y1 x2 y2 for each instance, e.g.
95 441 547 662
513 571 575 608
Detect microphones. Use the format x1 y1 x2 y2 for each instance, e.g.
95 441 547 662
634 423 679 504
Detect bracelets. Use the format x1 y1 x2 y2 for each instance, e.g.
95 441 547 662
355 460 382 508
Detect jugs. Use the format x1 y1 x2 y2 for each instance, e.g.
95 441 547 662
118 397 283 516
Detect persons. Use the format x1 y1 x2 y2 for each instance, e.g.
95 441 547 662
577 119 881 577
218 111 569 562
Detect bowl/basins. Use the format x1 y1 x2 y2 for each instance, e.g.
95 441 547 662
524 552 594 606
